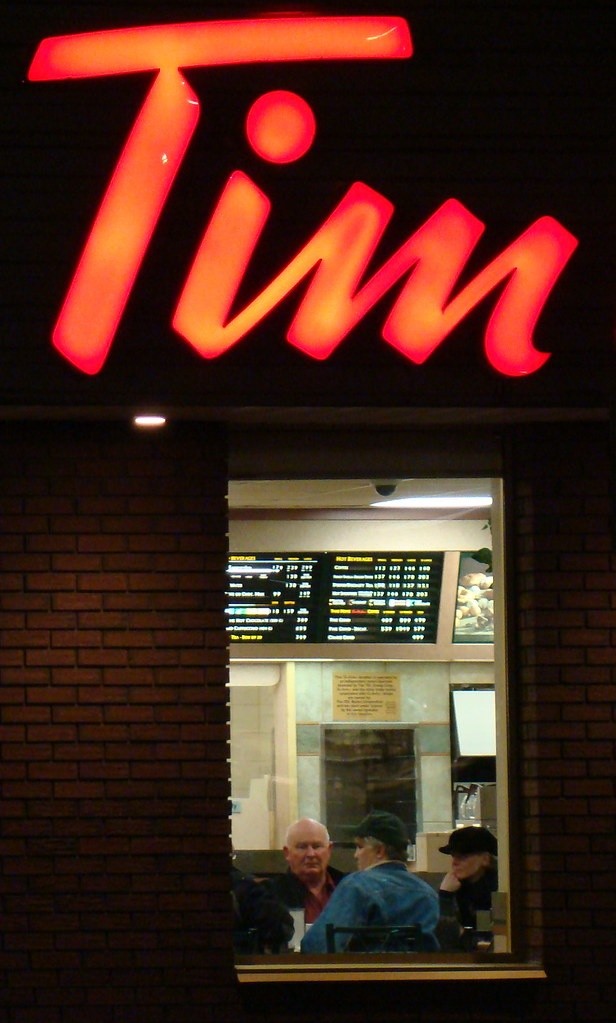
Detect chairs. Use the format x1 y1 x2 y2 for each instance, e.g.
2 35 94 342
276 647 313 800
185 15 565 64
326 920 422 952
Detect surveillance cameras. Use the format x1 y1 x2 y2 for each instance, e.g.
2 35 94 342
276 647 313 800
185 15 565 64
370 480 400 495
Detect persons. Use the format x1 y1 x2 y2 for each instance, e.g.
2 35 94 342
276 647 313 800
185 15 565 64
227 808 440 957
438 824 498 952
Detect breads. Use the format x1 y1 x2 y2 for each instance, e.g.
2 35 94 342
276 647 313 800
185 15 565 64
453 572 495 625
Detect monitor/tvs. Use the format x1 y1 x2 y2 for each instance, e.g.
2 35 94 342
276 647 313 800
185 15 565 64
450 682 497 762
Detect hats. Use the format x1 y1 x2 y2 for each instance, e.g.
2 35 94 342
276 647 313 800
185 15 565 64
343 811 408 849
439 826 498 856
